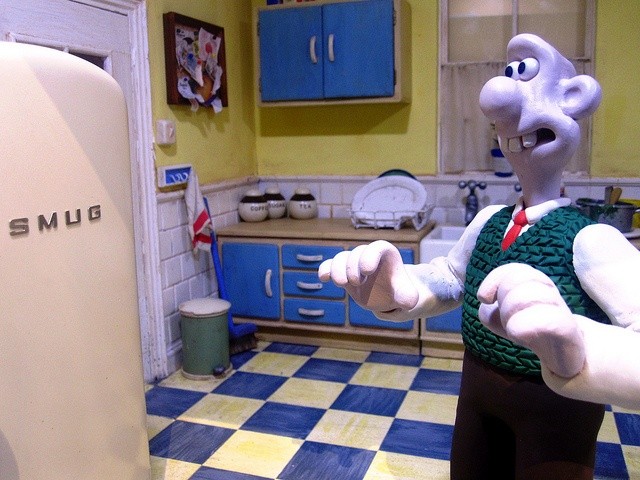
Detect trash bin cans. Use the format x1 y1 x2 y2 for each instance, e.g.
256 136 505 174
179 299 234 380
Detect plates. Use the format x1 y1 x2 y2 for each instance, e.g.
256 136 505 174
351 176 426 227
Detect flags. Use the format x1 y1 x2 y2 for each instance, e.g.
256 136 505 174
319 32 640 479
